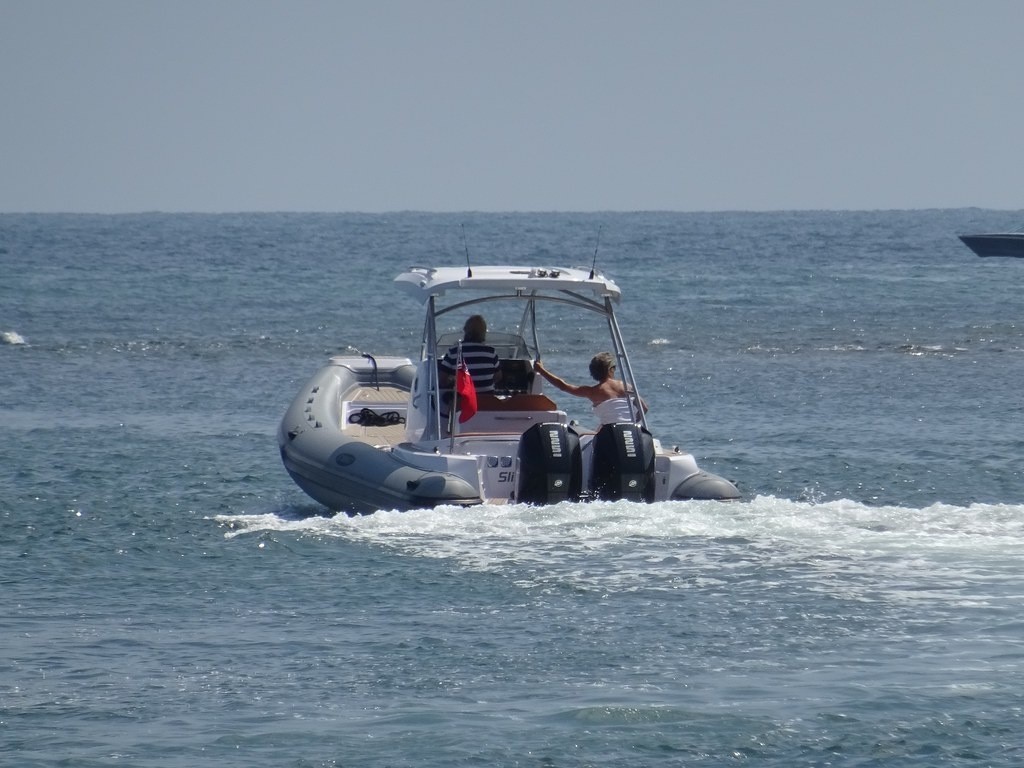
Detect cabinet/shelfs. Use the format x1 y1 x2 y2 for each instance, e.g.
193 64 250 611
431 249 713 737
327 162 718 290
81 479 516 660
447 392 567 435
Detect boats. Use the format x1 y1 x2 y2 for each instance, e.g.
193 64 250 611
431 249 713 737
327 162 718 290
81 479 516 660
958 232 1024 257
276 260 740 520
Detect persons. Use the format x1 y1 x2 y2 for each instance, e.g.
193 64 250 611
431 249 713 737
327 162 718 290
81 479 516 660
533 352 648 432
440 315 502 395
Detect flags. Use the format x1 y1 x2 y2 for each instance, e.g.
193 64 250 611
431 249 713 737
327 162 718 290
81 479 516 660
457 350 477 424
